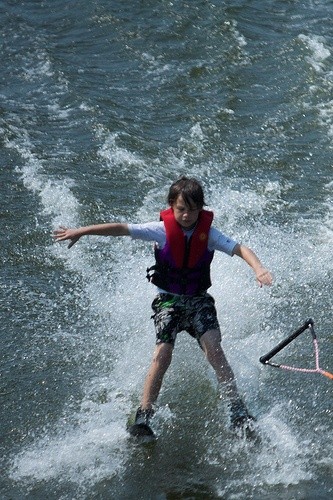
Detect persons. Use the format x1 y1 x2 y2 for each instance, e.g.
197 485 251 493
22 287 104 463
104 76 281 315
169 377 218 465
49 176 273 445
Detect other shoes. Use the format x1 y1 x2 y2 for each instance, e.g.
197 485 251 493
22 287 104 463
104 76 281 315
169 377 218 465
228 398 258 435
129 406 157 437
